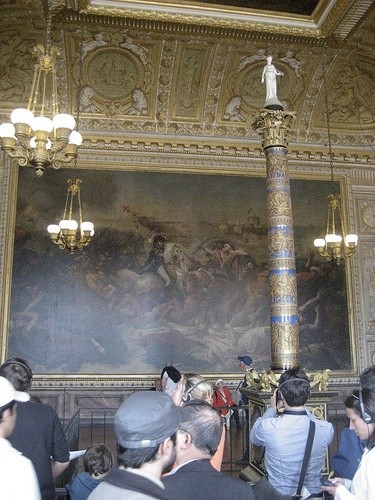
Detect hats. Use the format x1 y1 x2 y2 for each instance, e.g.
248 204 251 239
238 356 253 366
113 390 182 448
0 378 32 408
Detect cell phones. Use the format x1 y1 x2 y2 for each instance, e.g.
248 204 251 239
320 478 334 486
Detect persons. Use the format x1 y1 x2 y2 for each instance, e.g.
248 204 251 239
0 376 42 500
0 358 72 500
66 356 375 500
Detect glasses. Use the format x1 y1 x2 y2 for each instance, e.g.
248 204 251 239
184 399 214 409
348 392 360 400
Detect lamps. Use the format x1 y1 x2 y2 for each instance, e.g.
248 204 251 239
0 0 83 178
314 64 358 264
47 167 95 255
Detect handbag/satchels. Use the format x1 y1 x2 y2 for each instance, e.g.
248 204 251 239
254 476 303 499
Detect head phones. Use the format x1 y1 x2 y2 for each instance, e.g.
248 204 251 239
183 379 207 403
358 390 372 424
277 379 311 398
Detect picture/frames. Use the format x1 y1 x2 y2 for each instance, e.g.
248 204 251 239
0 160 358 382
